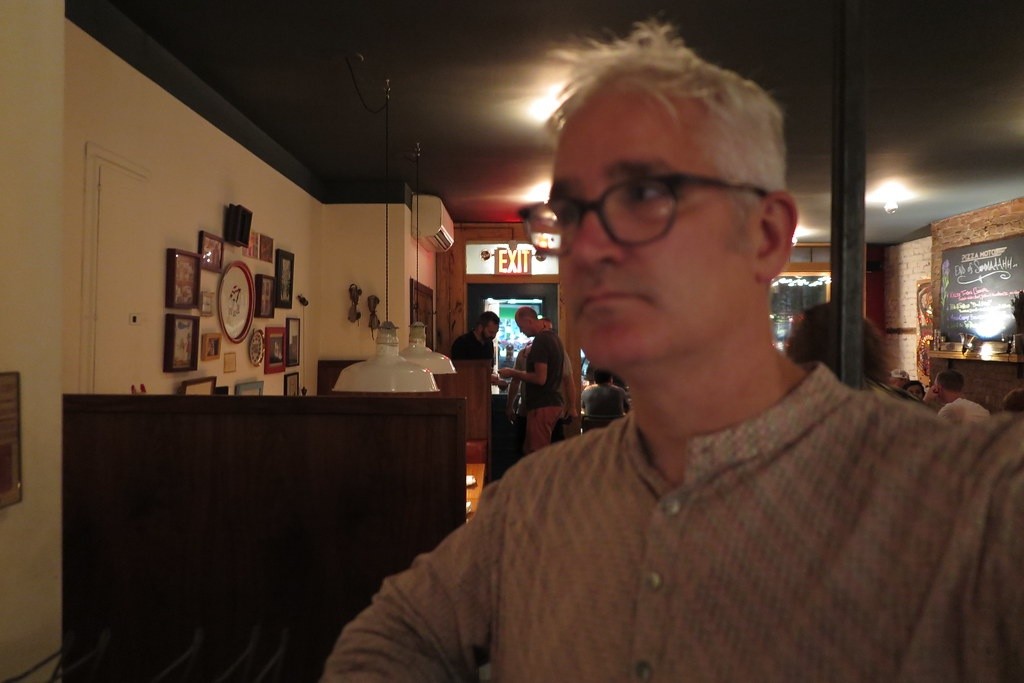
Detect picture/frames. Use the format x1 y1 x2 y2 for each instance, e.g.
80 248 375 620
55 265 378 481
235 381 264 395
254 274 276 319
198 230 224 274
275 248 295 309
201 333 222 360
181 376 217 395
0 371 23 510
284 372 299 396
201 291 214 317
165 247 201 310
286 318 300 367
264 327 286 375
163 314 200 373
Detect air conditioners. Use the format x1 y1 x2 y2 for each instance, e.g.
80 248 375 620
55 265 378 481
412 195 454 253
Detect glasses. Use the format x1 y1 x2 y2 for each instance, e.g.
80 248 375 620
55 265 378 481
517 174 768 255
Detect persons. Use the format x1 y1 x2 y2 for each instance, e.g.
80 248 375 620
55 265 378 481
325 21 1024 682
452 307 630 455
785 300 1024 422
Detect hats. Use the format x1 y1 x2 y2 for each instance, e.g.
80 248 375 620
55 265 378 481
891 369 909 380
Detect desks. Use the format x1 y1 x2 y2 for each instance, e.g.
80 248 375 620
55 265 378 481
466 464 485 522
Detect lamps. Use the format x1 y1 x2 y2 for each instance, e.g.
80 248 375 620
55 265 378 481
331 52 442 392
399 140 457 374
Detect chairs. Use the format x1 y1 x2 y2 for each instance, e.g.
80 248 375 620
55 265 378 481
582 415 622 434
